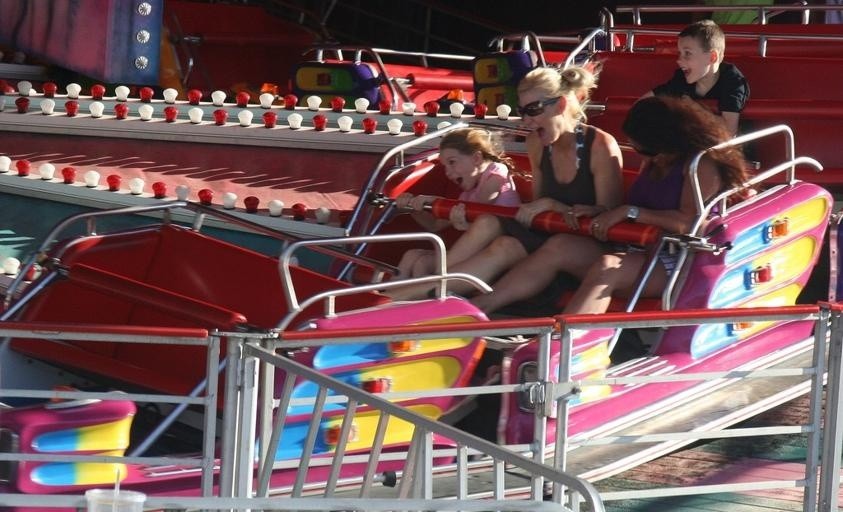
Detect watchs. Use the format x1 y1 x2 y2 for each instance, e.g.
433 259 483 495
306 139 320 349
626 205 640 222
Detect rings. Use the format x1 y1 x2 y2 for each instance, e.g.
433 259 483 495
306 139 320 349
593 223 599 227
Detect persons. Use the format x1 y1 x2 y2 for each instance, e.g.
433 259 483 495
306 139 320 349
633 18 757 170
467 95 748 315
383 65 624 301
390 126 523 280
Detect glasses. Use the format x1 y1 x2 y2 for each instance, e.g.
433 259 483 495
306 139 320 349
631 144 658 157
514 96 560 121
